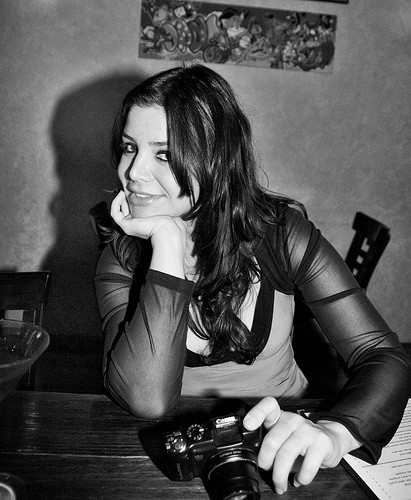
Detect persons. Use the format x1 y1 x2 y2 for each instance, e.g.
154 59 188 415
92 66 411 495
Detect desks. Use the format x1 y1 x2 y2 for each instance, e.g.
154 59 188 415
0 392 367 500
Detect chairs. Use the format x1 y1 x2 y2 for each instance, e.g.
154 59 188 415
0 272 50 392
345 212 390 291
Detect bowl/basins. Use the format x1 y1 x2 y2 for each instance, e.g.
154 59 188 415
0 319 49 404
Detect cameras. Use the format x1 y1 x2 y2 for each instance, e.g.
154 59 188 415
161 404 263 500
